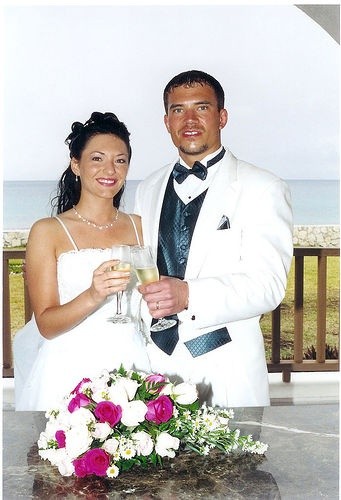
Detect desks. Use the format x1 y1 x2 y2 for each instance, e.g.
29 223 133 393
2 406 339 500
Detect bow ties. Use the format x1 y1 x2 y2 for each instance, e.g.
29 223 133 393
172 161 207 185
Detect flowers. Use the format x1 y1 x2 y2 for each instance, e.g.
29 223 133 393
37 362 268 479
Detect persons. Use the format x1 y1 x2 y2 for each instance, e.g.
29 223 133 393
16 112 152 412
131 69 294 407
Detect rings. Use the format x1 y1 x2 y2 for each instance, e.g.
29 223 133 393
156 302 159 309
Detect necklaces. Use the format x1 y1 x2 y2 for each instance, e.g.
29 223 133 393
73 205 118 229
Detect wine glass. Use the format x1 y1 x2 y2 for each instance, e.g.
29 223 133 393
131 245 177 332
108 244 132 323
